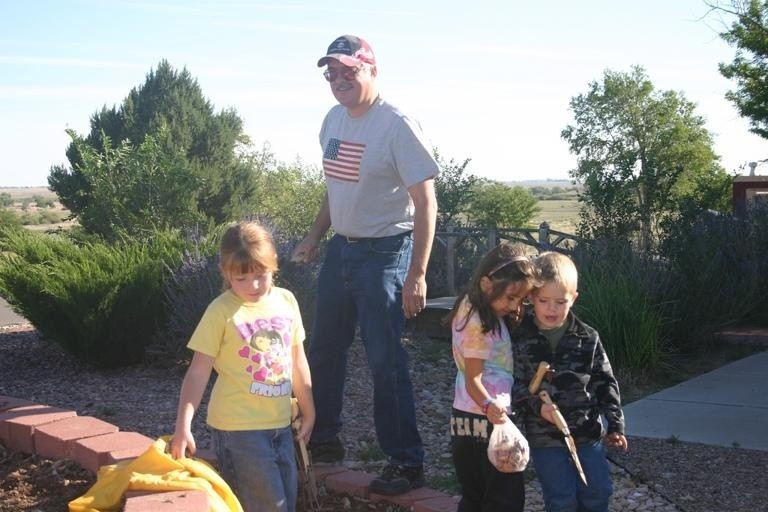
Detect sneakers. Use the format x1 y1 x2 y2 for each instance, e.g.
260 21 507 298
297 436 345 463
367 463 426 495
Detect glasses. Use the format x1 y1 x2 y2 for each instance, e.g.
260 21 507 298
324 67 357 81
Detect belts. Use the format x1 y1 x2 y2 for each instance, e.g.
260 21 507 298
347 236 359 244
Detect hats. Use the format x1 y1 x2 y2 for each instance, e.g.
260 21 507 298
318 34 377 68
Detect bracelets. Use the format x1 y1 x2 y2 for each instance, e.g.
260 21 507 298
480 399 492 413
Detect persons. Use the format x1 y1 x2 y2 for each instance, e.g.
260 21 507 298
165 221 316 512
509 250 628 512
452 244 546 511
291 35 441 495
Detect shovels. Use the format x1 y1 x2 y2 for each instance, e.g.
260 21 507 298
540 390 589 488
